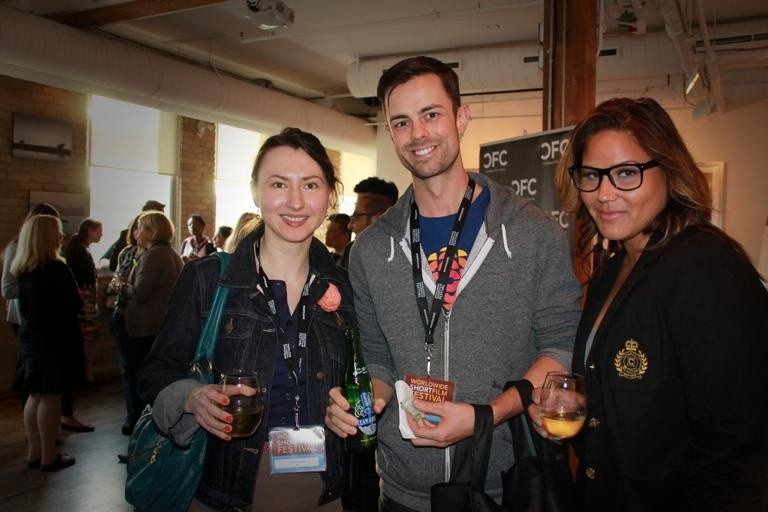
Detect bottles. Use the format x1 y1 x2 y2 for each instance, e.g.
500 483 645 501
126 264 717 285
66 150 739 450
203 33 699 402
340 327 380 449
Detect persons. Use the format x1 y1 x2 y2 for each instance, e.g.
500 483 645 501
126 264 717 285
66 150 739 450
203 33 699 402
137 126 359 512
225 212 261 254
323 55 586 512
528 97 768 511
100 198 234 464
325 176 398 512
1 199 104 472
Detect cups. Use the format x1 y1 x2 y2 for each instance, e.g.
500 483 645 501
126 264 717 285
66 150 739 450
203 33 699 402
218 368 263 439
541 370 588 440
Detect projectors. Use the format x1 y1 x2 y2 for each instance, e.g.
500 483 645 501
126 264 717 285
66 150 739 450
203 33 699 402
241 1 295 31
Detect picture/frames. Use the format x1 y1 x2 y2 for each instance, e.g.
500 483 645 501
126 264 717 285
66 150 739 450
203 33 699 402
695 161 725 232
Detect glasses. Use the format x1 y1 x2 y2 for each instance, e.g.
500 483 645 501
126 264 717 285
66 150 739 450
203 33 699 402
350 213 376 223
568 160 658 192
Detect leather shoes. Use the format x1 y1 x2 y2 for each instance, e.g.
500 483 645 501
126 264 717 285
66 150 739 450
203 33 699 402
28 454 75 472
61 422 94 431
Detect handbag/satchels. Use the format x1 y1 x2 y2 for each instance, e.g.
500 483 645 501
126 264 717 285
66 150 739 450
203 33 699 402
431 380 577 512
125 359 214 512
